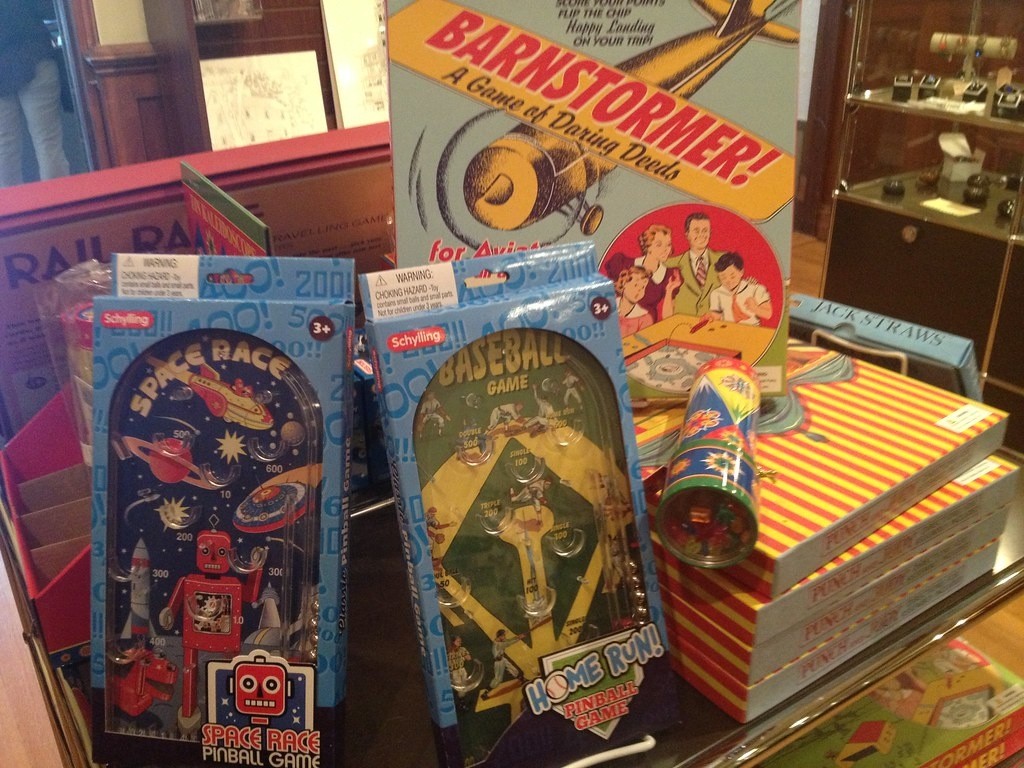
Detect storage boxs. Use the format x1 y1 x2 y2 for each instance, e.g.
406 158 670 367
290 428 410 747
0 0 1024 768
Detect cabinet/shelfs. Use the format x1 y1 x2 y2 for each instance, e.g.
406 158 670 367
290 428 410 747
820 0 1024 462
